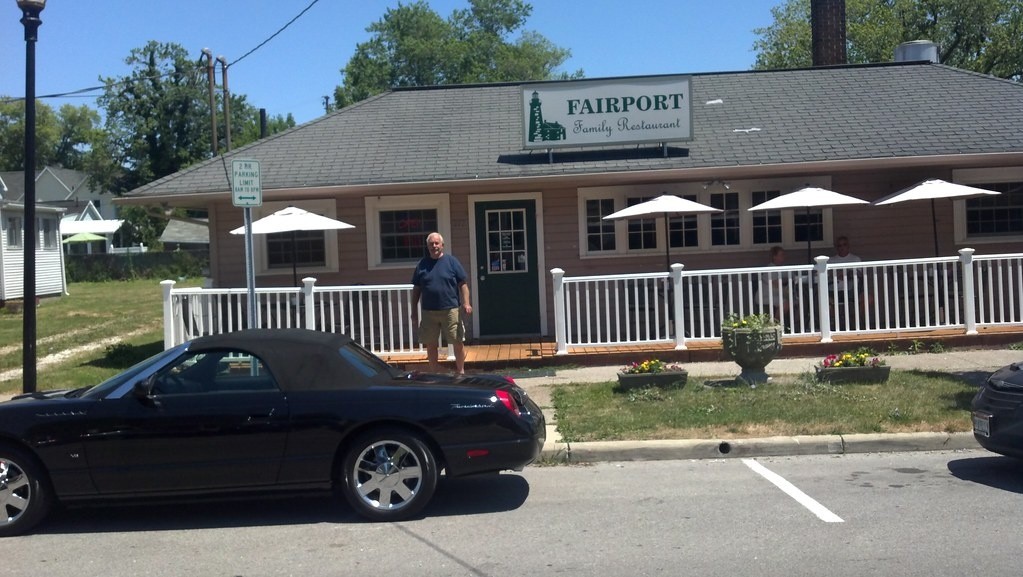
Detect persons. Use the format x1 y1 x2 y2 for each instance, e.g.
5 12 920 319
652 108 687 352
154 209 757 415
411 232 472 374
827 236 865 324
755 246 796 322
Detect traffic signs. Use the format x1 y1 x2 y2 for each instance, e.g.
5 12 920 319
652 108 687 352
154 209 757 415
231 159 262 207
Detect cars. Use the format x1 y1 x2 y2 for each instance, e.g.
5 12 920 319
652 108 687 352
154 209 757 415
0 328 547 537
969 360 1023 464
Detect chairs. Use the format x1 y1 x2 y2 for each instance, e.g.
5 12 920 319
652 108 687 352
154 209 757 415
329 283 373 337
622 279 656 341
181 294 217 337
684 269 717 337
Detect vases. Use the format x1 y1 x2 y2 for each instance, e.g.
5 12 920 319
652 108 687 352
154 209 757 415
722 325 780 386
617 369 688 390
814 365 891 385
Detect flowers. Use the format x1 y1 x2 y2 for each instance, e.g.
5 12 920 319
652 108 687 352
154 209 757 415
620 358 668 373
721 313 780 357
817 353 886 369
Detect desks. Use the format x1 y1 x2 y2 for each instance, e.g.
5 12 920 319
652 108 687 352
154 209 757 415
260 301 329 329
782 278 839 331
647 288 691 338
910 272 961 325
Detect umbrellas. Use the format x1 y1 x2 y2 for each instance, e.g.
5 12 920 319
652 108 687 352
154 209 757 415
602 195 725 339
871 176 1002 257
228 206 356 287
747 187 871 265
62 233 108 244
